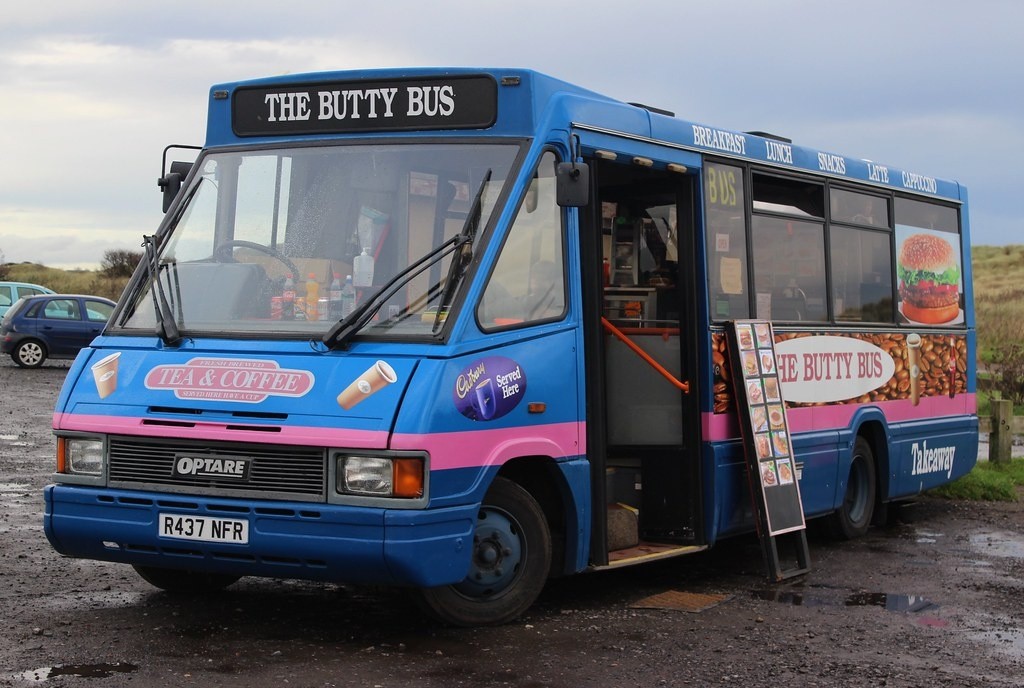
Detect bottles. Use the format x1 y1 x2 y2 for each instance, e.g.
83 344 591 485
306 274 318 320
284 274 295 317
341 279 354 319
785 279 799 300
331 274 342 322
354 248 374 287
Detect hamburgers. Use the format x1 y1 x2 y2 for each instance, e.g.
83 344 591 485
898 234 960 324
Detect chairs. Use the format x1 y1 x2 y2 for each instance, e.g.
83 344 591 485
68 304 74 318
278 159 393 275
73 303 80 319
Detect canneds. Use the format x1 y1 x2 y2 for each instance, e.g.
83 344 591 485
317 297 329 321
294 297 306 321
271 297 283 321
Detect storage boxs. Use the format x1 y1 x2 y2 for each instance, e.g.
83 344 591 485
237 255 359 305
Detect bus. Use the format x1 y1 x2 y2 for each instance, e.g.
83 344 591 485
44 67 979 628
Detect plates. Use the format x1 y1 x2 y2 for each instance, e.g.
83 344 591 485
769 411 784 426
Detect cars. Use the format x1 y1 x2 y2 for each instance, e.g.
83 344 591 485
0 282 106 324
0 294 118 368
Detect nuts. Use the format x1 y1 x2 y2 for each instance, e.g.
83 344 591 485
709 331 967 414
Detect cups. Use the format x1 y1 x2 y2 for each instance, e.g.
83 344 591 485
91 352 121 398
906 334 921 406
471 379 496 418
336 360 397 410
